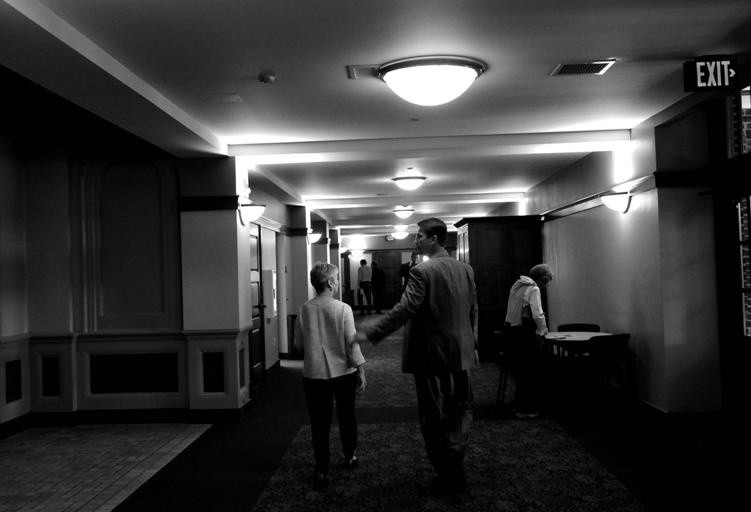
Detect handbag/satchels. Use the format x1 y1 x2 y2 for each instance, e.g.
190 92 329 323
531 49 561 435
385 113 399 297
522 304 535 328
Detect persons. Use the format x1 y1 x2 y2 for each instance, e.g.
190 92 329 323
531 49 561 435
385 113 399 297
370 260 384 314
357 258 372 316
397 250 419 290
345 216 479 503
292 262 367 483
501 262 553 423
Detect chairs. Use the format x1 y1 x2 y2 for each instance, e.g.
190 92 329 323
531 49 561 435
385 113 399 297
490 321 631 421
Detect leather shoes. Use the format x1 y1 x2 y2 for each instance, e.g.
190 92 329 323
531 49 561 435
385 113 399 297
315 471 325 480
349 455 358 464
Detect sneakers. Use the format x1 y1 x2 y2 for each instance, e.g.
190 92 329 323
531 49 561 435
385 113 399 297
514 407 539 419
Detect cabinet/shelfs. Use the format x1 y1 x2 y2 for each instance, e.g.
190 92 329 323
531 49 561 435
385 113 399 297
455 213 543 363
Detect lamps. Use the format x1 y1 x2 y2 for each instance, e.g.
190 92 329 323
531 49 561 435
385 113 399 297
239 203 267 226
599 192 632 214
373 53 489 108
307 233 322 244
390 175 428 219
